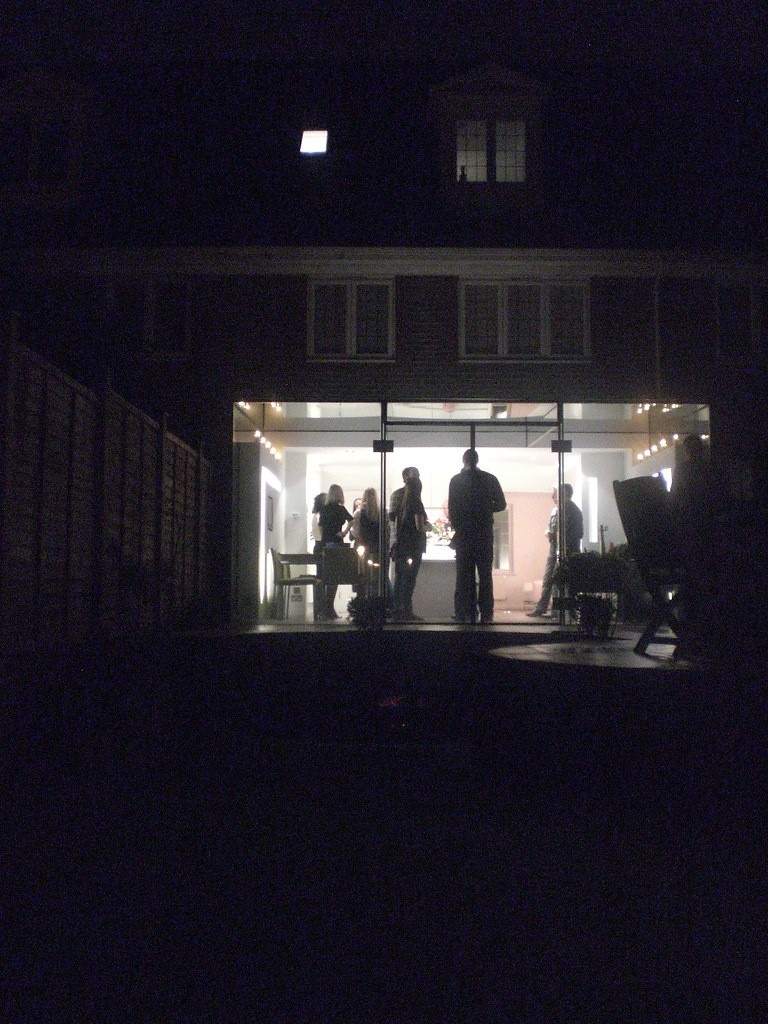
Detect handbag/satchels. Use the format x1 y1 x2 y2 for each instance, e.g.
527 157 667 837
414 513 432 532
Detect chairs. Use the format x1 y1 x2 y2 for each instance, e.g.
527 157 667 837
269 549 323 625
614 472 716 658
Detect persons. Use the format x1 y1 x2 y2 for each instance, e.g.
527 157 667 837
669 435 707 491
299 466 434 621
445 451 506 622
527 484 584 617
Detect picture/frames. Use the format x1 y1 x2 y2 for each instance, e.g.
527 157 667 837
265 493 273 531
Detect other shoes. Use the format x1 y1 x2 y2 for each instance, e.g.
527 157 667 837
400 611 425 621
525 607 547 617
